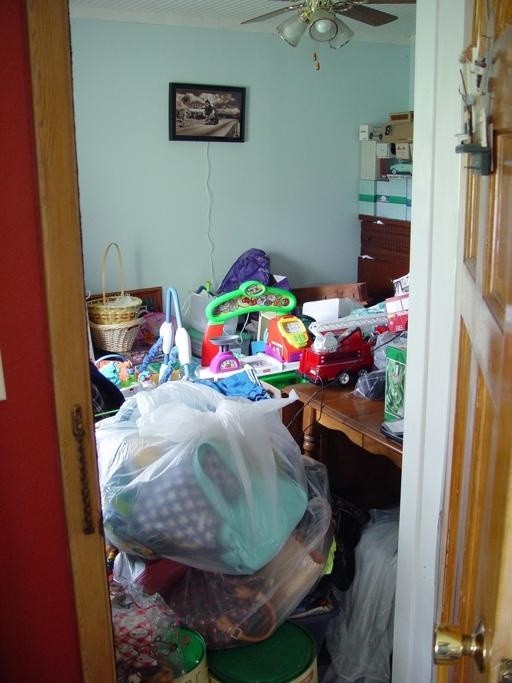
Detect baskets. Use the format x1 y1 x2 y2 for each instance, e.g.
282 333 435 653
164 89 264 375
87 243 150 353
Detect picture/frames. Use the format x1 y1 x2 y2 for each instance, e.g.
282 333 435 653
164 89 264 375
167 79 247 144
86 286 164 320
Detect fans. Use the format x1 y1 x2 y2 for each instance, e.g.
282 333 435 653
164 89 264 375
239 0 416 28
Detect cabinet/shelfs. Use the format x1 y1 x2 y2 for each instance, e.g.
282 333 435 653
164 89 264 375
353 214 413 306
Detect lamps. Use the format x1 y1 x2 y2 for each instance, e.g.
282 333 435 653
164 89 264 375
277 1 354 71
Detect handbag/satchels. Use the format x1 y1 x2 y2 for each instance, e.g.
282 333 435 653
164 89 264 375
192 439 309 572
173 569 278 646
255 498 332 615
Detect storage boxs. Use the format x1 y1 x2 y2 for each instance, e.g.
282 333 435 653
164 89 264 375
289 282 369 307
355 107 415 224
301 296 364 323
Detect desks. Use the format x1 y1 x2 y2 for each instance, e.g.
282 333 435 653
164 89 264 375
281 382 404 467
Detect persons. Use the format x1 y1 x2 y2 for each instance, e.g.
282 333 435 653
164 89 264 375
201 99 218 126
